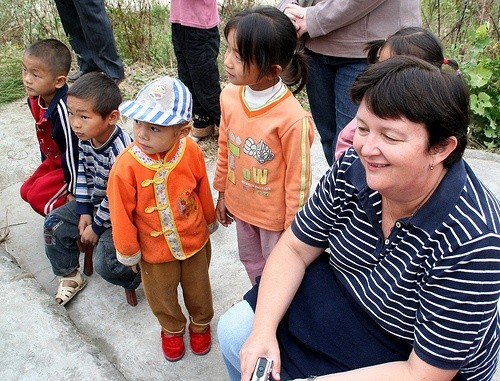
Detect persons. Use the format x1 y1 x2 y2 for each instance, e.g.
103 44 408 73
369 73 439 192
168 1 221 142
274 0 422 164
107 75 218 362
336 26 461 164
215 55 500 381
43 73 141 304
54 1 125 85
215 5 315 288
20 38 78 217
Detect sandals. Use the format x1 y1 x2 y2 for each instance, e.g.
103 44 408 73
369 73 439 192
54 271 87 306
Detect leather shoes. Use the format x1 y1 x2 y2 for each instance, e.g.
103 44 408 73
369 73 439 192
69 71 125 86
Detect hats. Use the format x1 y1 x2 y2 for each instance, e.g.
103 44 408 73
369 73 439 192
118 76 192 126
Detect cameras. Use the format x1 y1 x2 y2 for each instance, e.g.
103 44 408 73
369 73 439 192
251 357 273 381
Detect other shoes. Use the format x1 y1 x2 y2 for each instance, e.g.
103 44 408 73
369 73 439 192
187 132 201 143
161 324 211 361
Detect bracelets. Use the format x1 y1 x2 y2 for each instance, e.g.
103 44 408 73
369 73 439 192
307 376 317 381
217 198 224 202
